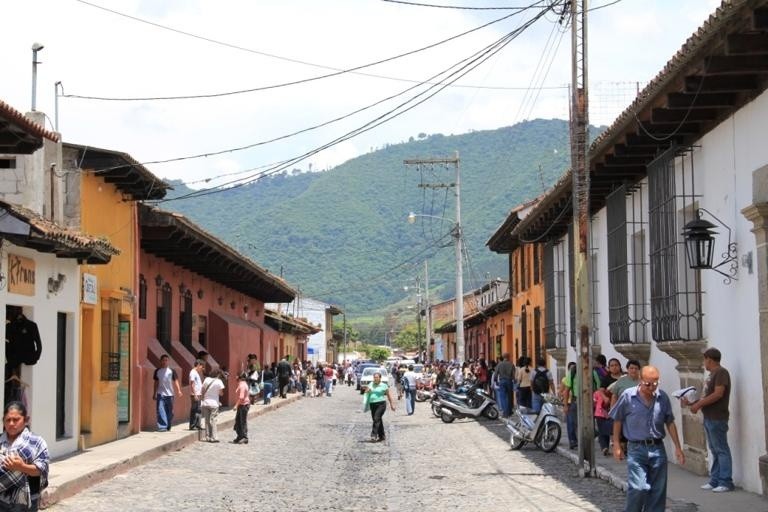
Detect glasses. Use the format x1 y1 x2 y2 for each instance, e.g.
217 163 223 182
642 381 658 386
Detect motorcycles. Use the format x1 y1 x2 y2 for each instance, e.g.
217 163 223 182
504 392 565 453
414 379 500 422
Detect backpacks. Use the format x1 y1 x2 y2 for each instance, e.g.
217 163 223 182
532 368 550 394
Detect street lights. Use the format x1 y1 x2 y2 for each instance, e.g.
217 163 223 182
407 209 465 371
403 286 431 362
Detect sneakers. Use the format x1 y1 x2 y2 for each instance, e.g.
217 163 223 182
700 483 713 489
712 485 728 492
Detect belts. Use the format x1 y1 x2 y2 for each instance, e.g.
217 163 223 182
634 439 659 444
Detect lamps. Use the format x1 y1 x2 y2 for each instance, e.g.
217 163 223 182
678 207 739 286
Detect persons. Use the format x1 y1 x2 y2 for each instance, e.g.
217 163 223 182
0 348 735 512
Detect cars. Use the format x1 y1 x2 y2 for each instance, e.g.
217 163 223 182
351 352 433 390
